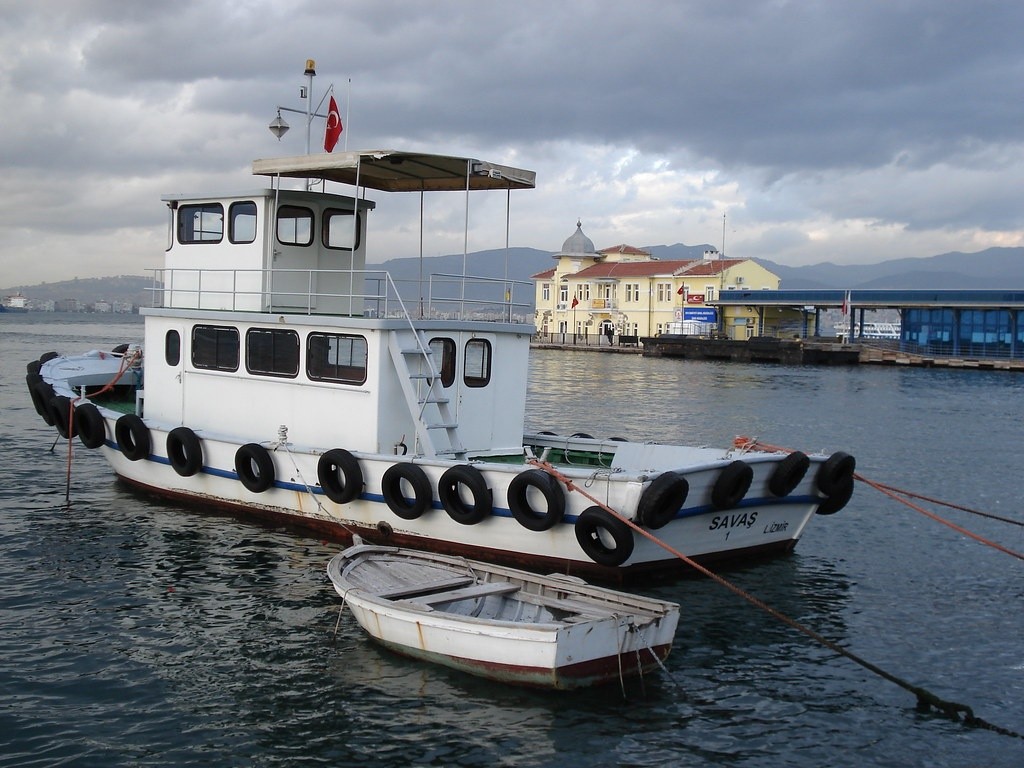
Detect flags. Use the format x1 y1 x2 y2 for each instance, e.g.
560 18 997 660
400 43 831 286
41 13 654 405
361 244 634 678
571 294 579 309
320 95 344 154
841 291 852 317
676 285 684 296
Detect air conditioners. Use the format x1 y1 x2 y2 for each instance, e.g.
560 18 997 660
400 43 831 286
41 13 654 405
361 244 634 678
709 322 717 329
746 317 754 324
736 276 743 284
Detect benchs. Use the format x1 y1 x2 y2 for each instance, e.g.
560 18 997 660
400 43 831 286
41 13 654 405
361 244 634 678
618 335 638 346
398 581 520 604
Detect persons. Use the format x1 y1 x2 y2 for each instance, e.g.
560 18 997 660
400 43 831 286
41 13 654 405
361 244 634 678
606 328 614 347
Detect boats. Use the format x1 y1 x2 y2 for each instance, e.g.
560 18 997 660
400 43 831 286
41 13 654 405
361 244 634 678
27 58 857 590
327 543 680 693
